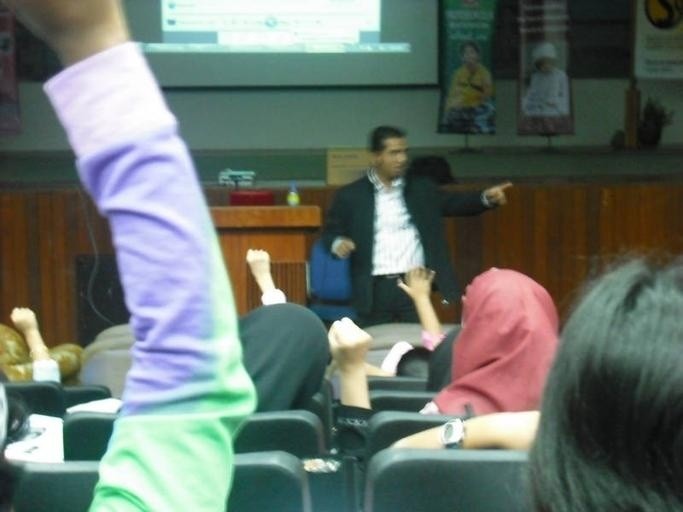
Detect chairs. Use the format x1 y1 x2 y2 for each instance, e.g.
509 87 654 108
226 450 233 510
0 366 537 511
303 235 367 323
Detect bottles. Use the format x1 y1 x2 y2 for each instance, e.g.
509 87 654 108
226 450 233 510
286 179 300 205
624 79 643 150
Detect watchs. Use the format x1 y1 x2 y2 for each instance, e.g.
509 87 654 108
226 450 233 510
441 417 465 449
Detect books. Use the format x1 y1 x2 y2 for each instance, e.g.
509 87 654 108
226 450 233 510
364 264 462 391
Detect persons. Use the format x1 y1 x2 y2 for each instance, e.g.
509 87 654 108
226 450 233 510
0 0 257 512
238 248 373 454
521 43 571 119
421 268 559 416
0 303 62 385
440 41 494 130
387 412 544 451
528 252 682 512
319 124 513 328
